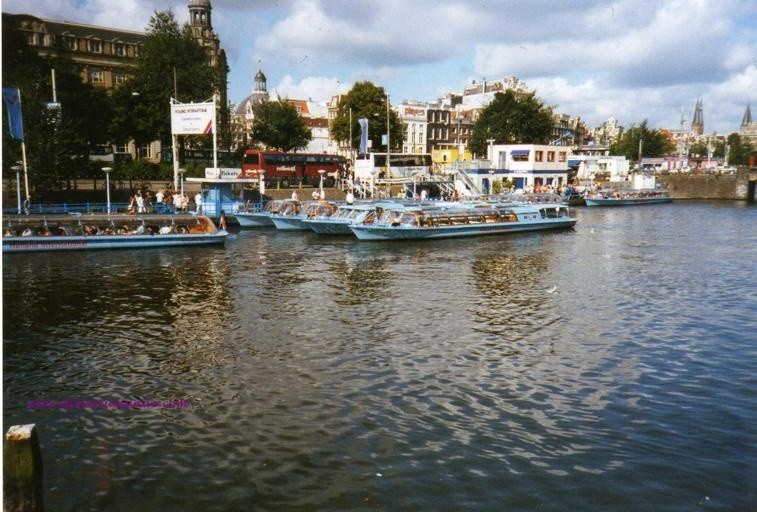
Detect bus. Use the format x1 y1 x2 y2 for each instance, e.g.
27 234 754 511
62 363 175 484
353 152 434 186
241 149 352 188
156 147 242 178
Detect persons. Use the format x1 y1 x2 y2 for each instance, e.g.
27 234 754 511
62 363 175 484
245 195 336 219
333 169 341 188
22 195 33 215
126 187 190 215
525 181 668 201
311 189 320 200
415 188 459 203
345 189 354 211
193 191 203 213
6 217 189 237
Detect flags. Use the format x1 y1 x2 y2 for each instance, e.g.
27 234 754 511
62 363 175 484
218 215 227 230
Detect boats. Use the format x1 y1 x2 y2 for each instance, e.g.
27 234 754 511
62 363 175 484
583 185 674 207
2 214 228 251
232 198 577 241
